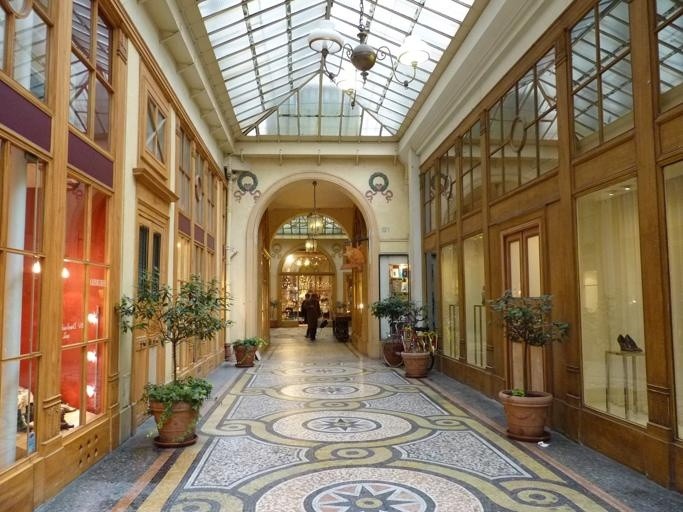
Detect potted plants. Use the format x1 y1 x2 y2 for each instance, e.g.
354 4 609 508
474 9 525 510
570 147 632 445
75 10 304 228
371 296 431 378
488 289 568 443
115 266 259 448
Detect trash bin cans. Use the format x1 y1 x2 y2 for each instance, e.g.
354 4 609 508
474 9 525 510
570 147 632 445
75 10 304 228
336 317 349 341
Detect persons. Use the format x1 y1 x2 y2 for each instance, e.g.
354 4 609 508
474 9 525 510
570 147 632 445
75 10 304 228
300 294 311 338
303 292 320 341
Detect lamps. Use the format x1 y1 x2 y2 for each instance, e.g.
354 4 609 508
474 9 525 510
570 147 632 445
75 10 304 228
308 1 430 110
